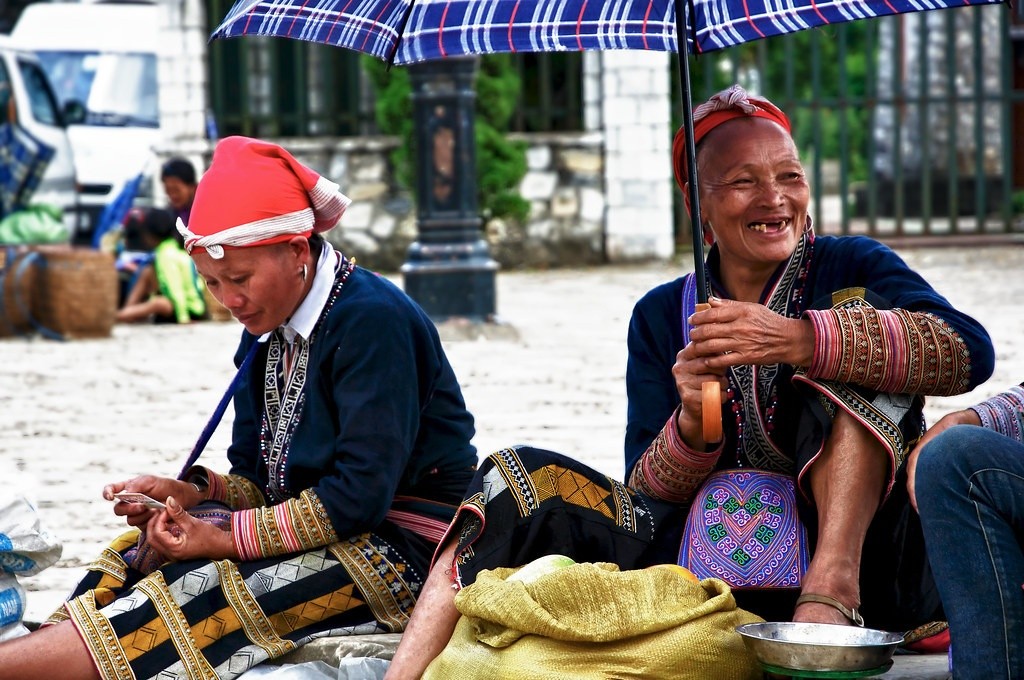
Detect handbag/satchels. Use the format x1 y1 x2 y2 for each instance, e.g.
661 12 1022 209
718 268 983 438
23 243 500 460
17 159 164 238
678 467 810 592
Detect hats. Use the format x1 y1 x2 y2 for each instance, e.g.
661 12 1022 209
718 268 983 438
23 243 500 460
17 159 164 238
671 85 790 248
175 136 352 259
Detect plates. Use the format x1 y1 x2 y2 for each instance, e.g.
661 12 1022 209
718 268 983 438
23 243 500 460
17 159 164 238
731 620 907 672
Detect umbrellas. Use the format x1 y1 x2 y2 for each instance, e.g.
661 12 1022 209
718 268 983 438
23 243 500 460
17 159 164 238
207 0 1004 445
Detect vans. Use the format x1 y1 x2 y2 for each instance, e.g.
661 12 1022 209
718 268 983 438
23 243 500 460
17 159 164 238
10 1 169 252
1 34 91 254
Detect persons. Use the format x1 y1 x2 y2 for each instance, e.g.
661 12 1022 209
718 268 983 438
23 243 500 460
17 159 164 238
112 156 211 325
0 135 483 680
381 84 999 680
905 380 1024 680
